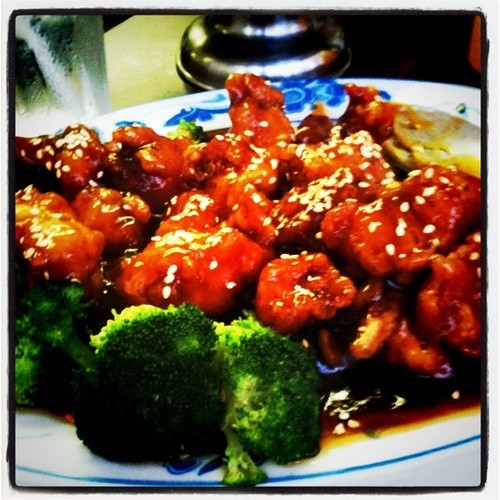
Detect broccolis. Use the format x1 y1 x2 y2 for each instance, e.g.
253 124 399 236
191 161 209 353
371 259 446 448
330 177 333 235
14 256 322 488
167 118 210 144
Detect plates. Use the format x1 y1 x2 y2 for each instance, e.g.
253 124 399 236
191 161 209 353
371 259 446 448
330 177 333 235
14 78 484 487
